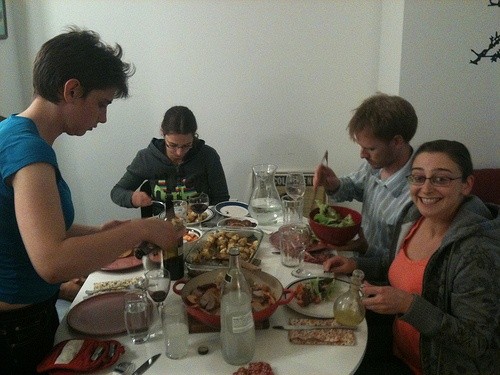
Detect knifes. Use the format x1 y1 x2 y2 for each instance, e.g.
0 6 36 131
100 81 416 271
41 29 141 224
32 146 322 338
131 353 162 375
273 325 358 330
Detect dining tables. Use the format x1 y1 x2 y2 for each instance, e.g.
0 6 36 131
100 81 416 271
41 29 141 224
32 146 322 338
53 206 368 375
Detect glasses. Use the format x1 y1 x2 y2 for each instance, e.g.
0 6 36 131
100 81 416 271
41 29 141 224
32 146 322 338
164 140 193 149
405 174 464 186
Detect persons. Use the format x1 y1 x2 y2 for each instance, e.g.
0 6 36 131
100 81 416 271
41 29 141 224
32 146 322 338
323 140 500 375
0 28 179 375
111 106 231 219
313 93 418 287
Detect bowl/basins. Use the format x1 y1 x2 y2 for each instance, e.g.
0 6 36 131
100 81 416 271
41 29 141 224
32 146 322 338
307 206 362 246
217 217 259 229
164 200 187 216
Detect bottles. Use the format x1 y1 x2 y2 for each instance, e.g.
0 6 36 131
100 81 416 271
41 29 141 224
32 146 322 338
219 249 255 365
163 193 179 222
333 270 366 329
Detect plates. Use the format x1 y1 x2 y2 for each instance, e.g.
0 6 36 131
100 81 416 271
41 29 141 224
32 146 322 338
214 200 250 217
183 227 202 244
271 228 327 252
281 275 364 319
101 255 142 271
160 208 213 224
67 290 156 337
183 227 264 276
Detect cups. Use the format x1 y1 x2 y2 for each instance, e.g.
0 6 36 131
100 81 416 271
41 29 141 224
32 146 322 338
278 224 303 267
162 302 189 360
123 289 151 343
280 195 304 226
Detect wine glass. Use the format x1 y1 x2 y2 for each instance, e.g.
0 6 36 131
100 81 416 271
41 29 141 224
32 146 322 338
284 173 306 207
188 194 209 230
289 222 312 278
145 269 171 333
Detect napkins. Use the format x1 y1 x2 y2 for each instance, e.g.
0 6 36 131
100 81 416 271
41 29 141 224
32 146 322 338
289 319 356 346
233 362 273 375
269 224 329 264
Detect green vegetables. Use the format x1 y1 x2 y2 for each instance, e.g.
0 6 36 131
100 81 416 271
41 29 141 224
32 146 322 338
302 200 355 242
304 277 337 301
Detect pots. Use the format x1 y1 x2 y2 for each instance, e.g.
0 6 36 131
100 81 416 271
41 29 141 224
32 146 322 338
172 266 295 327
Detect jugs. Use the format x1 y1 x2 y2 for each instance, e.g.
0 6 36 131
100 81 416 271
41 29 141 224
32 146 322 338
249 161 283 235
